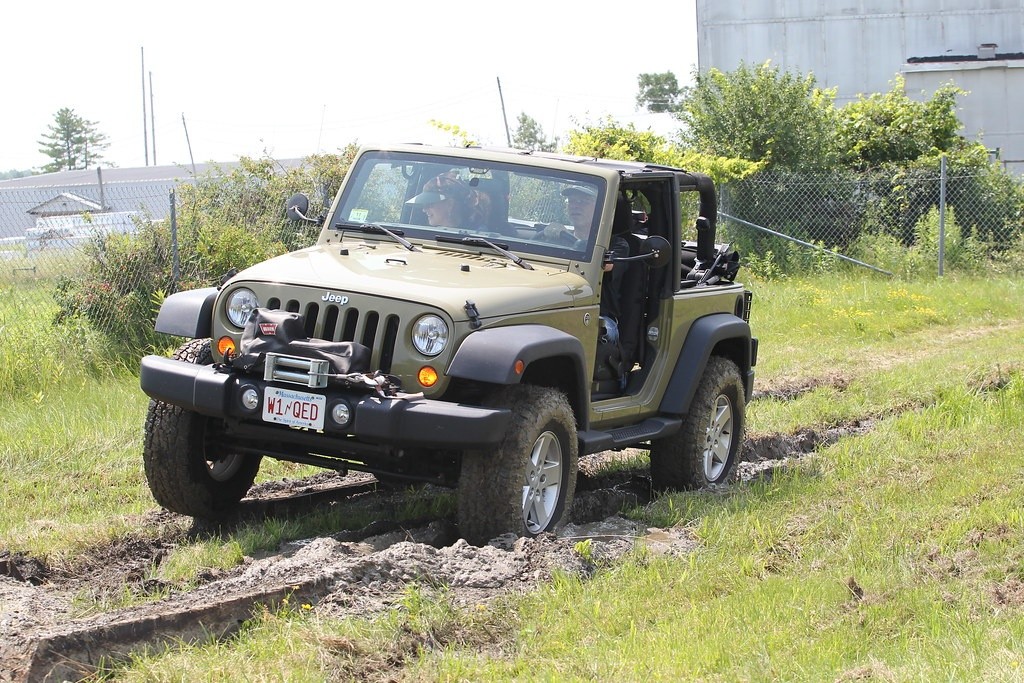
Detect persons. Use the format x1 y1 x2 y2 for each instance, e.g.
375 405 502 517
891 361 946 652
544 184 629 271
405 172 491 231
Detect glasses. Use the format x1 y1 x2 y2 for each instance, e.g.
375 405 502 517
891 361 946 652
564 198 594 206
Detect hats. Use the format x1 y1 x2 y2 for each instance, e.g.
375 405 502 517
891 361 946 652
561 184 597 197
405 191 448 207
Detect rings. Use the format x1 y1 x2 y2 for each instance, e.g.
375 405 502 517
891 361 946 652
552 226 557 231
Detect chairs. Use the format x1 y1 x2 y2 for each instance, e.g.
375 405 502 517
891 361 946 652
474 177 513 233
616 192 652 371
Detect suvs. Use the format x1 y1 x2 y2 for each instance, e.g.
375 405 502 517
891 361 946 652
140 140 760 548
